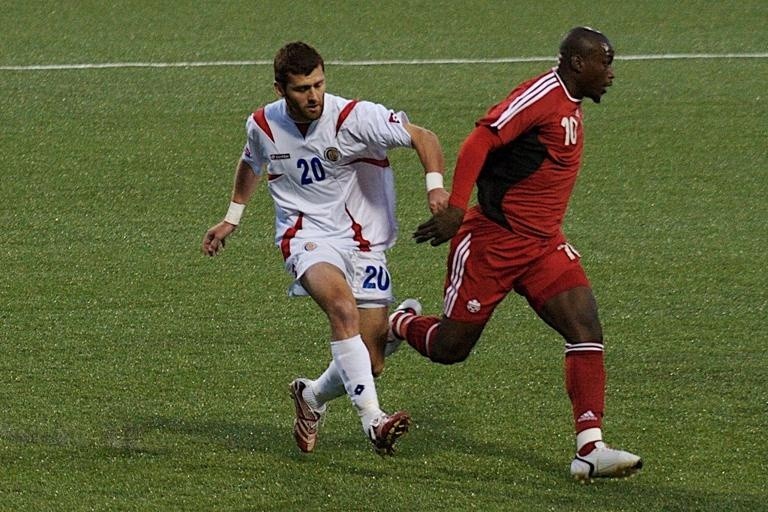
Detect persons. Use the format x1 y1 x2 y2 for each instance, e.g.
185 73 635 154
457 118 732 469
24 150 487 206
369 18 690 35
382 26 644 483
201 40 452 451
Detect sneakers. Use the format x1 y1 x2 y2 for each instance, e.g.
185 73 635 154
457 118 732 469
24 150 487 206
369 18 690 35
378 297 424 358
569 441 644 482
288 378 323 455
371 410 412 457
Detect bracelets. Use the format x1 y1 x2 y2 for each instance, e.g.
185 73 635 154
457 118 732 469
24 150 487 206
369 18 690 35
223 200 247 228
423 172 445 194
